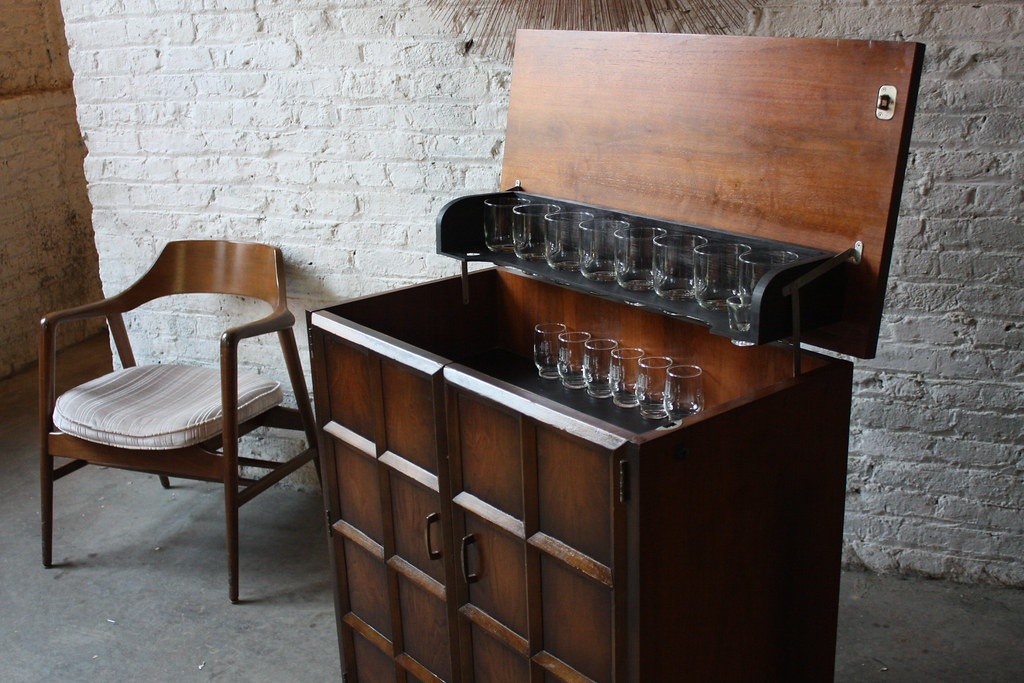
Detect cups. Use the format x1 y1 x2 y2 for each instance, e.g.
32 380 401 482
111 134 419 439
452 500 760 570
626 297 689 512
582 339 618 398
579 218 630 281
483 198 531 252
544 212 594 270
663 365 704 420
635 357 673 419
512 204 561 262
653 234 708 302
727 294 757 347
692 242 751 310
557 332 592 389
738 249 798 308
533 323 567 380
613 226 667 291
609 348 645 408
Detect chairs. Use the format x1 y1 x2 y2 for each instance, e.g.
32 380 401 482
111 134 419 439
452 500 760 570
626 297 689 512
38 238 318 603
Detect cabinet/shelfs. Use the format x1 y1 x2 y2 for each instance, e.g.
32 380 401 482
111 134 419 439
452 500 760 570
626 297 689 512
306 30 928 683
